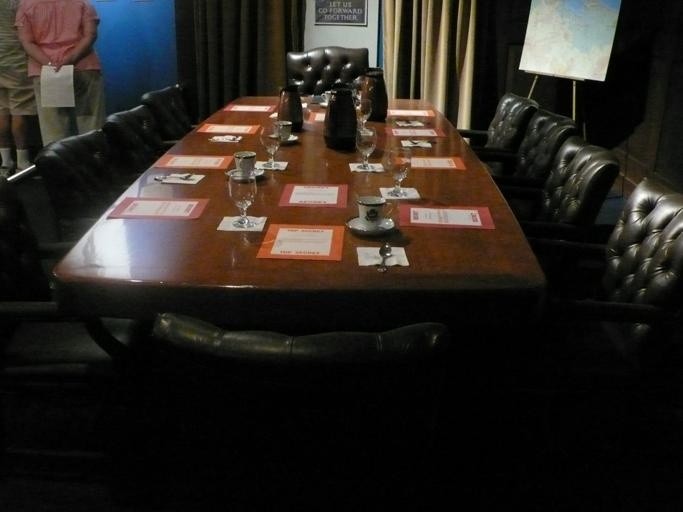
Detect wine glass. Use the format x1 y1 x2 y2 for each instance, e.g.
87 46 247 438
259 133 283 168
355 126 379 171
382 146 410 198
226 169 260 229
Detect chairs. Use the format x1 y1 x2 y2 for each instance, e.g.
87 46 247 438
35 129 143 213
0 164 141 481
458 93 538 150
105 105 167 166
147 315 450 512
140 85 194 139
485 109 578 183
506 177 683 433
500 136 619 223
286 48 369 95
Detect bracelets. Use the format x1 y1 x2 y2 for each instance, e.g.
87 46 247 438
48 61 52 66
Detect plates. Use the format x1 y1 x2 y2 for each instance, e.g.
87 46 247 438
344 216 396 237
220 168 264 183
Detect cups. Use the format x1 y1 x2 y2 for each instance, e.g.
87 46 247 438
356 195 394 229
267 85 375 145
361 68 390 124
233 151 258 177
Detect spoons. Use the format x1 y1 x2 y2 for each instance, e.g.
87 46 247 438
407 137 436 147
205 134 239 144
153 171 195 183
376 241 393 274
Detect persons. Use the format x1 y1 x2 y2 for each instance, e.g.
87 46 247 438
13 0 107 147
0 0 38 179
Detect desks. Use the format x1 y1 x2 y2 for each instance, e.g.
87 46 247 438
48 96 549 295
